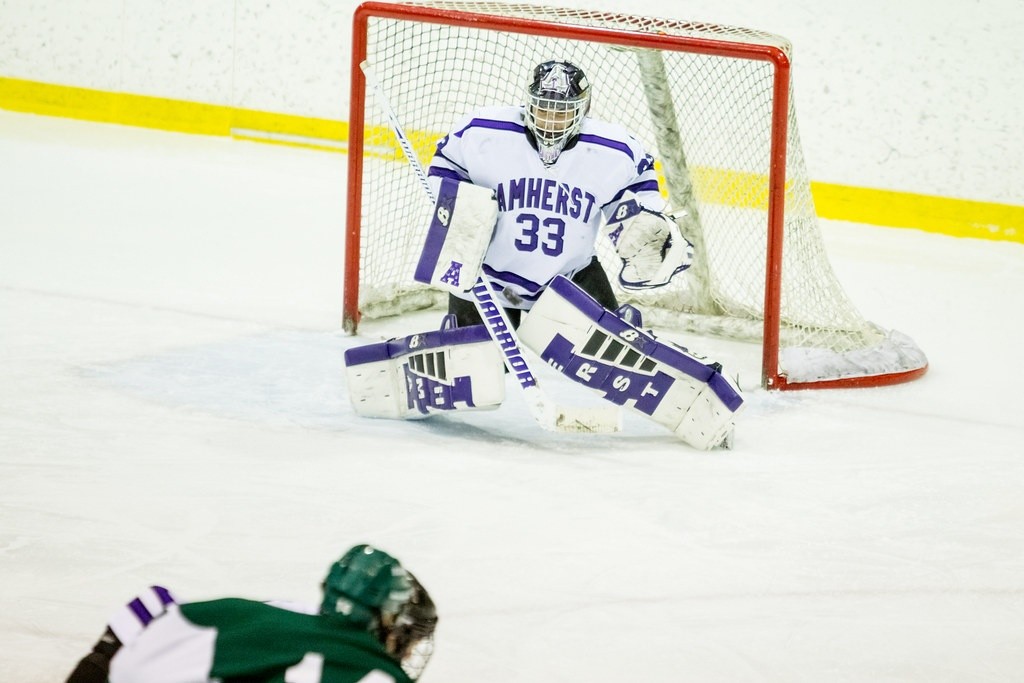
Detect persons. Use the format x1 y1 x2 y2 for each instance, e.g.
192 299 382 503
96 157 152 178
344 61 744 451
65 545 439 683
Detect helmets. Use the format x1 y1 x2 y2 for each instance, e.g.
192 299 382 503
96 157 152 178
323 545 436 683
523 60 592 166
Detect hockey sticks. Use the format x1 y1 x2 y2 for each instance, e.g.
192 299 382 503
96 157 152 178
358 60 559 426
